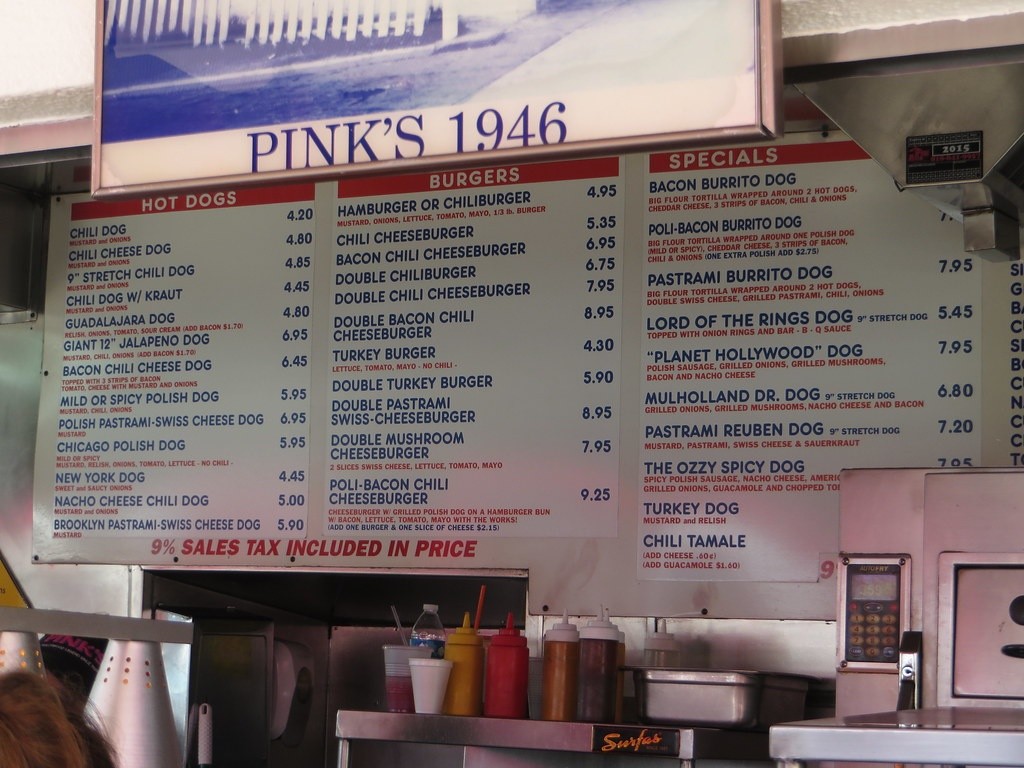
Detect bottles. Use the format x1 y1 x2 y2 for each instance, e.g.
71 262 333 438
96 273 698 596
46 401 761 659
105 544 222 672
444 611 484 717
541 608 579 723
485 613 530 719
581 604 619 724
605 608 625 724
644 619 680 668
409 603 446 659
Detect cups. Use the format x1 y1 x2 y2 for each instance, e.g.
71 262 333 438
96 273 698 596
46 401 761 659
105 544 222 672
408 657 453 715
382 643 432 713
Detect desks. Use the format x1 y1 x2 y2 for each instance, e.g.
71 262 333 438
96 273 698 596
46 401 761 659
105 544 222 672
335 708 770 768
768 707 1024 768
